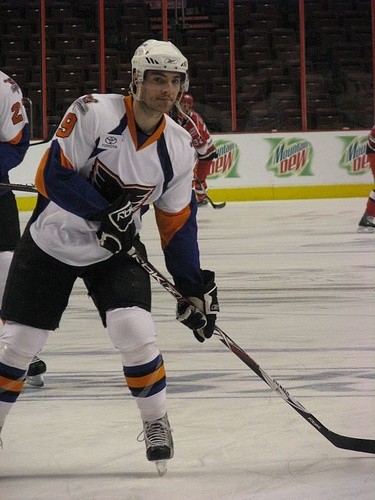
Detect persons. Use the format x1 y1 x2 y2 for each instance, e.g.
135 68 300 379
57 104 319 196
177 95 217 206
357 125 375 233
0 39 219 477
0 70 46 387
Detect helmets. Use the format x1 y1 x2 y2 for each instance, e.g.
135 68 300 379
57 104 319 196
128 39 189 104
179 94 194 105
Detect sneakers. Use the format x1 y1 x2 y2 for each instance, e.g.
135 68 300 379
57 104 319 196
197 199 209 208
357 214 375 233
27 355 47 387
137 411 175 476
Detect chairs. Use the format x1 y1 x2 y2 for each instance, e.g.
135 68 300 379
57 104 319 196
0 0 375 139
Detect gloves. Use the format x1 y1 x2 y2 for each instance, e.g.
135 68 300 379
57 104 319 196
175 270 220 343
96 199 136 255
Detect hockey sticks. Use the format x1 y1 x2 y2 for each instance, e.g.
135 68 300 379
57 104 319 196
0 182 39 192
196 176 226 210
125 245 375 454
